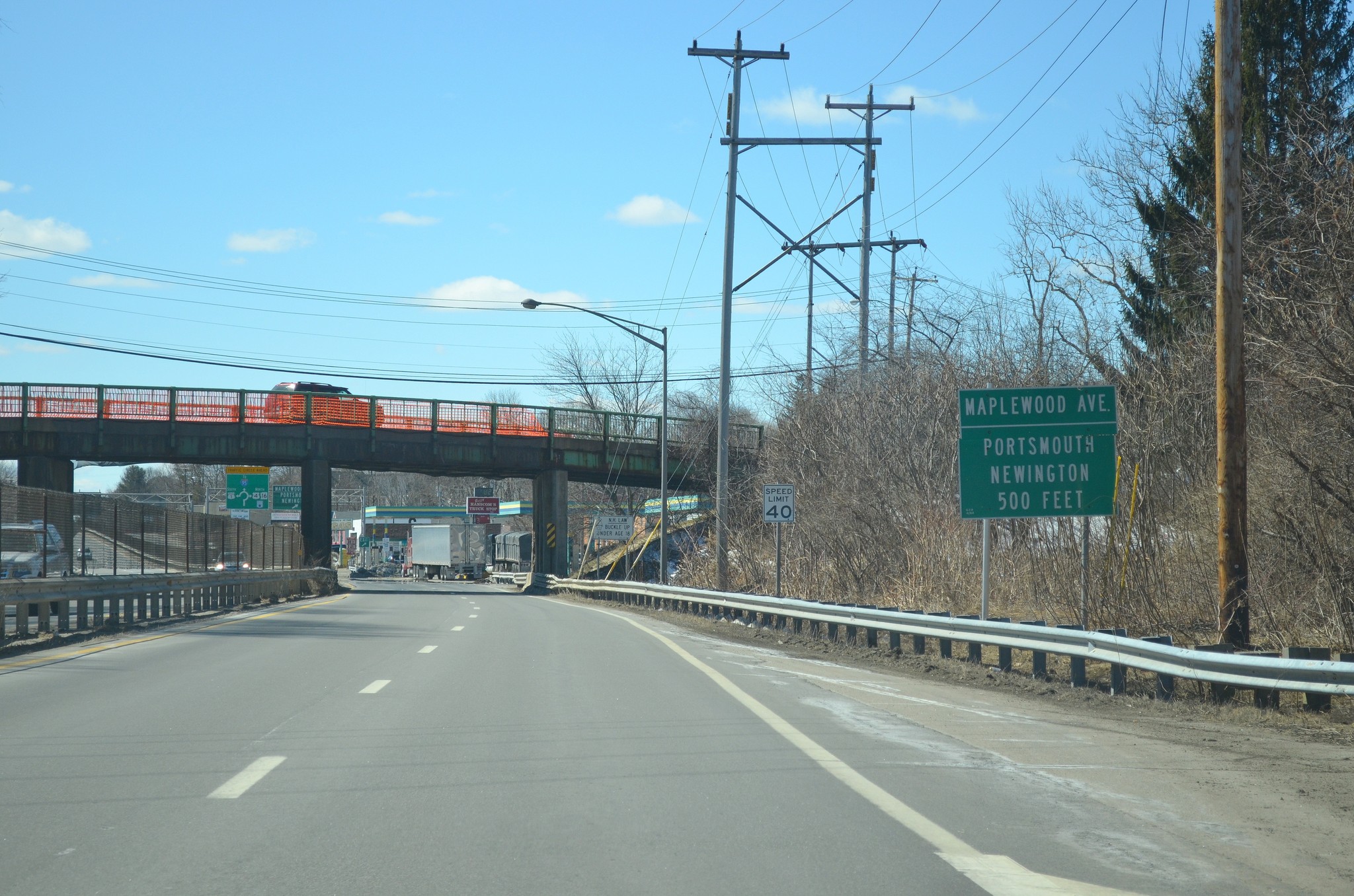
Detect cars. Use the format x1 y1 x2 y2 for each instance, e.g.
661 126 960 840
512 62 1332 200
73 515 82 527
387 555 404 564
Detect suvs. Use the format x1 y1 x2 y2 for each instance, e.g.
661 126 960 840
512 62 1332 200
212 550 250 572
0 519 71 615
264 381 385 427
76 547 92 561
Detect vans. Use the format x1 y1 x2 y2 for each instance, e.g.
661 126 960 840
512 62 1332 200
331 544 346 554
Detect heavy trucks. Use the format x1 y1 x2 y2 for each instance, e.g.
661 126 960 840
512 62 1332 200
401 523 487 581
487 531 532 587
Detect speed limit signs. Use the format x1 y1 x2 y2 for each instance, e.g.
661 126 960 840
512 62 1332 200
763 484 795 522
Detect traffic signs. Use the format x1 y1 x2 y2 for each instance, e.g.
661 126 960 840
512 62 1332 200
359 537 370 547
225 466 270 510
272 485 302 510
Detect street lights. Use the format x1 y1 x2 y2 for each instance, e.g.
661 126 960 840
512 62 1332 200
520 299 667 585
849 299 915 366
